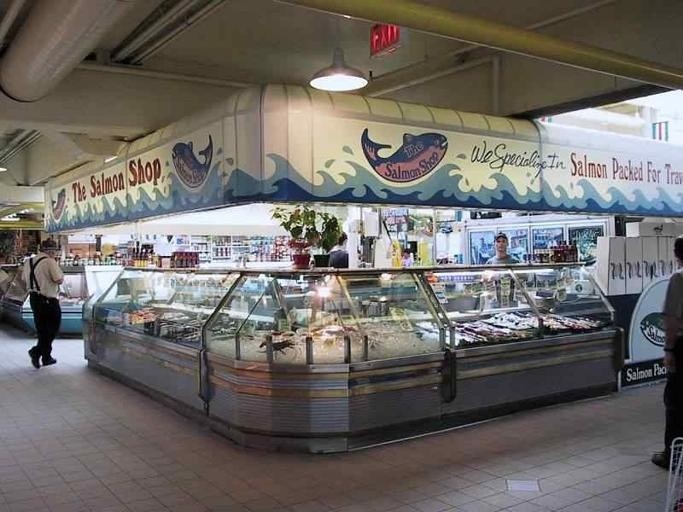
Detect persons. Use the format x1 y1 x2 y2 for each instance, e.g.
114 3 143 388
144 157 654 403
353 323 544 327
400 248 415 267
24 238 65 369
483 230 525 308
328 231 348 269
648 235 682 475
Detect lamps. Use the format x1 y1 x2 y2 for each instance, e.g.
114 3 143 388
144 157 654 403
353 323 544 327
309 14 368 93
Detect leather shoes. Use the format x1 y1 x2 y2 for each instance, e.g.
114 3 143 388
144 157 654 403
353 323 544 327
42 358 56 365
29 349 40 368
652 454 682 474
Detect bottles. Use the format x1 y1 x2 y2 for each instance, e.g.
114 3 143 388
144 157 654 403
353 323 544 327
131 241 149 267
171 250 196 268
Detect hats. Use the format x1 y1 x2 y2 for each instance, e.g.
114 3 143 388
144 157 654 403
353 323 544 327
494 233 506 241
40 241 59 250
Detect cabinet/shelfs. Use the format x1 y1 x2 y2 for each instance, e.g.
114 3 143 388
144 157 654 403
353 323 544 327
131 233 294 266
81 268 622 453
4 264 123 336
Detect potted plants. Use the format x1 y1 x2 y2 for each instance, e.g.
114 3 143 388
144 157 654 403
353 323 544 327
310 210 341 270
269 203 321 271
